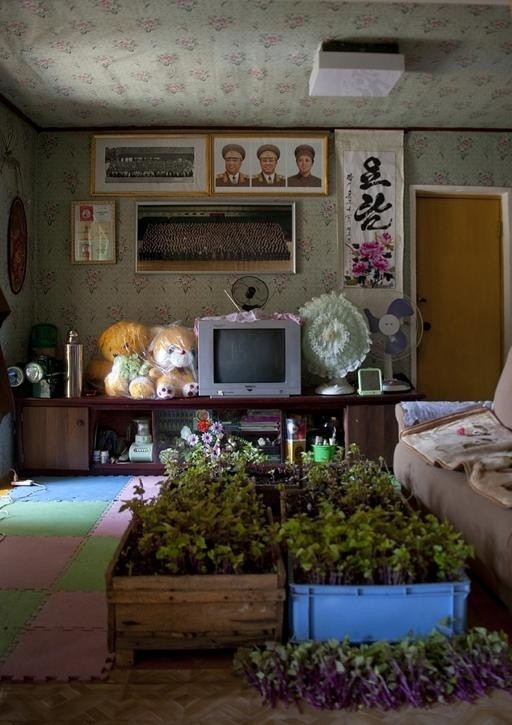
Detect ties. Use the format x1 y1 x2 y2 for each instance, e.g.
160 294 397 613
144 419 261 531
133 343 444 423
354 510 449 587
232 176 236 183
268 176 272 183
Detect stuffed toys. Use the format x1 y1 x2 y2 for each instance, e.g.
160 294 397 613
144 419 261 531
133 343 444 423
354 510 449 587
82 320 156 400
149 325 199 400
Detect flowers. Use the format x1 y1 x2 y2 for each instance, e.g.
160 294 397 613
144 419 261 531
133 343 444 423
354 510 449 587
181 418 224 456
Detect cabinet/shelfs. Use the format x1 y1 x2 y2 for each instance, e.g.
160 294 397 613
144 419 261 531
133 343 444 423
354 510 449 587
10 395 427 475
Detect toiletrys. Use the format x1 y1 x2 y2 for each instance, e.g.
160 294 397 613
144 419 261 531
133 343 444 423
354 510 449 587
329 426 336 445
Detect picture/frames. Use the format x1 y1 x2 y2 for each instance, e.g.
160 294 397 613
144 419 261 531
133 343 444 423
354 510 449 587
211 133 329 196
135 202 296 273
91 134 211 196
71 201 116 263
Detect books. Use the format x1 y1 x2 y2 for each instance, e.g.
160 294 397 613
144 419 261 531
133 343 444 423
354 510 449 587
215 407 280 432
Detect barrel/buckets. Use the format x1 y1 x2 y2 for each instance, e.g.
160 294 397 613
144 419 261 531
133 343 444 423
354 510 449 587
313 444 335 463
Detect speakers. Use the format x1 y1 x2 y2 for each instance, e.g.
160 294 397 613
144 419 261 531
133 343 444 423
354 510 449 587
357 368 382 395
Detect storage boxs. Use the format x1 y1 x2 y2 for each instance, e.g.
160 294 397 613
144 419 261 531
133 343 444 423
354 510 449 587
312 443 338 463
280 487 421 523
288 568 472 644
104 518 286 654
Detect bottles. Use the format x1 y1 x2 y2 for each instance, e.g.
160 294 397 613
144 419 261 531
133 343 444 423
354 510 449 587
65 329 85 398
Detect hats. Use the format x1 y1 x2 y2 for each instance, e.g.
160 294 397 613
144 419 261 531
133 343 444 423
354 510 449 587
257 144 281 160
295 145 315 161
222 144 245 160
268 151 270 152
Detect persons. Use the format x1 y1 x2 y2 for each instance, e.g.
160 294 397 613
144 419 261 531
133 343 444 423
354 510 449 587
251 144 286 187
287 144 321 187
215 143 250 187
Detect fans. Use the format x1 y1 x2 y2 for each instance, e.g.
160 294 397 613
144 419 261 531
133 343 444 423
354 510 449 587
232 276 269 310
359 289 423 392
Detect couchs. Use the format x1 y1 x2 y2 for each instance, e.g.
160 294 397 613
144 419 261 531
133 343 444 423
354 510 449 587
394 347 511 599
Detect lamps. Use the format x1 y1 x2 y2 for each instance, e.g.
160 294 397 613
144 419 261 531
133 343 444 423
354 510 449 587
307 41 405 98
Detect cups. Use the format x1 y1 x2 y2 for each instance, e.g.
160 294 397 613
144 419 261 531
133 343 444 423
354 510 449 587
310 444 337 464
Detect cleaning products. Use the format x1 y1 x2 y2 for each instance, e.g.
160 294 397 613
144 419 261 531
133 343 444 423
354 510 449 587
314 436 323 445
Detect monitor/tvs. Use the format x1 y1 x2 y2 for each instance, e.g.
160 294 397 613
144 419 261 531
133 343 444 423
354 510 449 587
198 319 302 398
